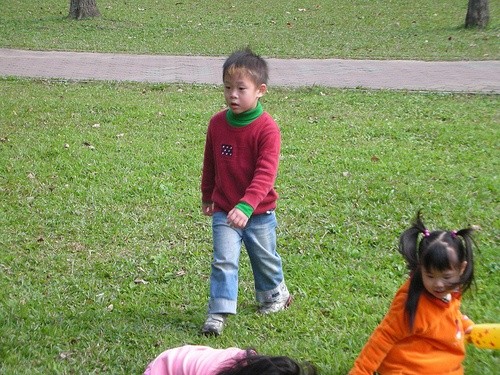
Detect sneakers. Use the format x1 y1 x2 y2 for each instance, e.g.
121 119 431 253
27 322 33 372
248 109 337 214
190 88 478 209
202 314 224 335
260 285 290 313
